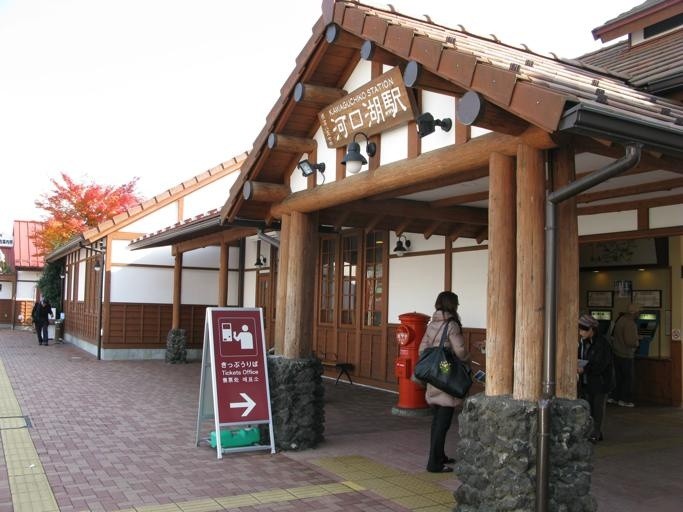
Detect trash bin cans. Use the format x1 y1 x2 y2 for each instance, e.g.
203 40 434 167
55 320 65 343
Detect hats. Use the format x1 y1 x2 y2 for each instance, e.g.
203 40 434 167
577 313 599 328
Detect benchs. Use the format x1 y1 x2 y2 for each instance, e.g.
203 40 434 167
320 359 355 386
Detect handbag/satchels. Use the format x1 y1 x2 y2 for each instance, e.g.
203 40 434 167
414 344 473 399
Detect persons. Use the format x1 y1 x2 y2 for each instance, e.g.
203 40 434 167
578 314 616 442
608 304 644 408
414 291 473 473
32 299 53 346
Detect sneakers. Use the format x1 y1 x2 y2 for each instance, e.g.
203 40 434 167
607 397 635 408
427 455 456 472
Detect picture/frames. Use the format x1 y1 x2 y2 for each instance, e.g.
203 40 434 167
587 290 614 307
578 237 669 269
632 289 662 309
